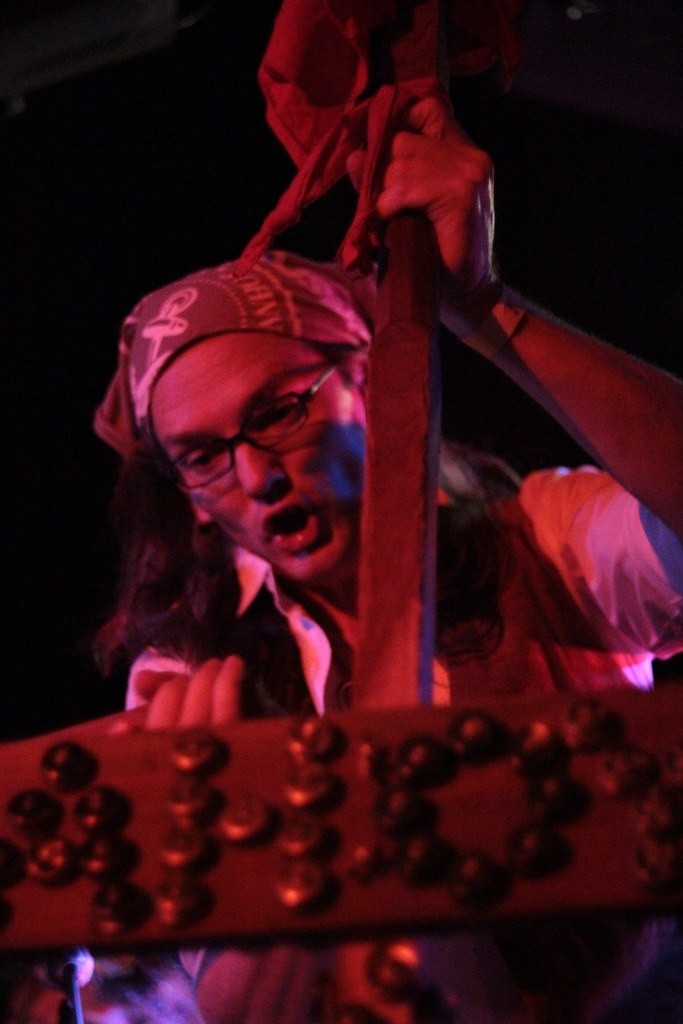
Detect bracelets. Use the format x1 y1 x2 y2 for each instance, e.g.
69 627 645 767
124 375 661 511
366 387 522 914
461 281 527 363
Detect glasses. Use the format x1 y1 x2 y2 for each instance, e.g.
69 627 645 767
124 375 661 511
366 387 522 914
164 366 338 489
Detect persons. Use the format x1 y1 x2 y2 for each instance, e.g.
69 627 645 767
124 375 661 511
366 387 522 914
14 97 682 1024
